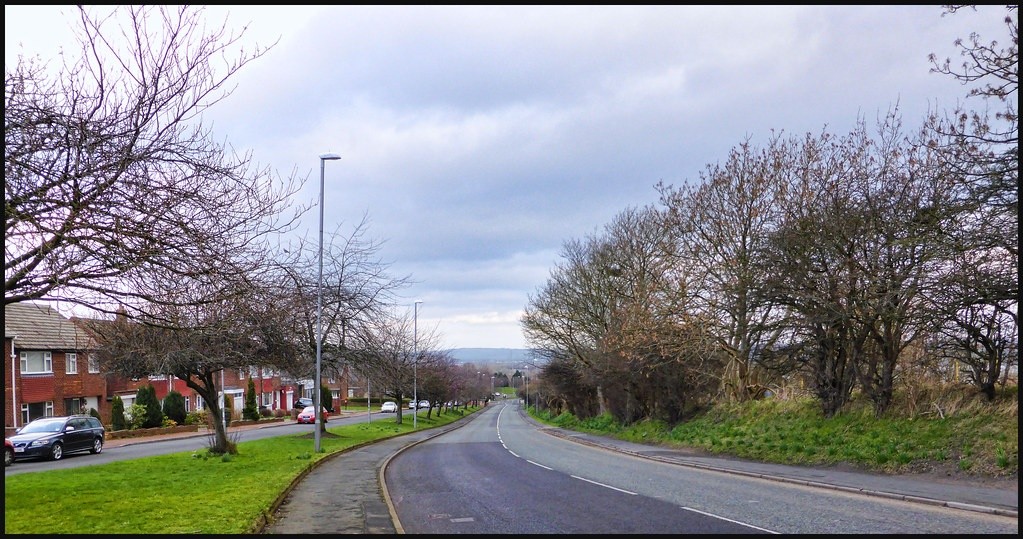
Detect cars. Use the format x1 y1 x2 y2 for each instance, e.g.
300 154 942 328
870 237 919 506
408 400 413 409
381 401 398 413
295 398 312 410
297 405 330 424
418 400 430 409
444 400 458 407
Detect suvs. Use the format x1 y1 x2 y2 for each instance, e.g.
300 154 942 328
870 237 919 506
7 413 105 461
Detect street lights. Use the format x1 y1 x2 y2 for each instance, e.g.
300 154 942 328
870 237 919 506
524 364 529 410
314 152 342 451
414 301 424 428
